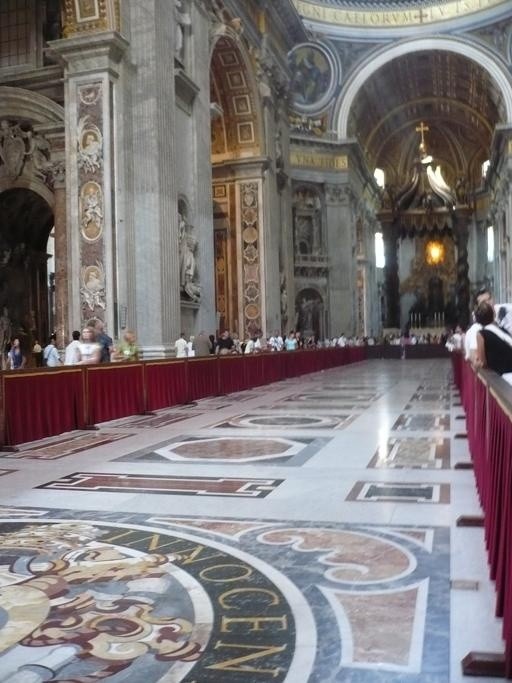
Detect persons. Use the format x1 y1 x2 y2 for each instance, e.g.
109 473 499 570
5 318 142 371
377 332 452 347
21 131 47 183
172 328 376 357
84 188 102 228
86 273 105 311
445 289 512 377
82 135 100 169
